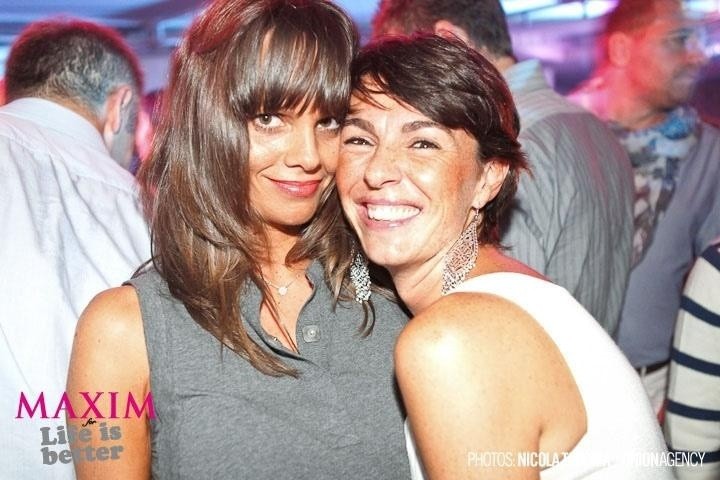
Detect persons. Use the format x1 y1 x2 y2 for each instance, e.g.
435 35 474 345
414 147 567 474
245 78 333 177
336 38 680 480
375 0 633 345
559 2 720 414
661 244 719 480
1 16 164 480
132 91 168 205
63 0 413 479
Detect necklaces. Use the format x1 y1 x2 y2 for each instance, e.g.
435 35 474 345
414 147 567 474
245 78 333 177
251 262 308 295
